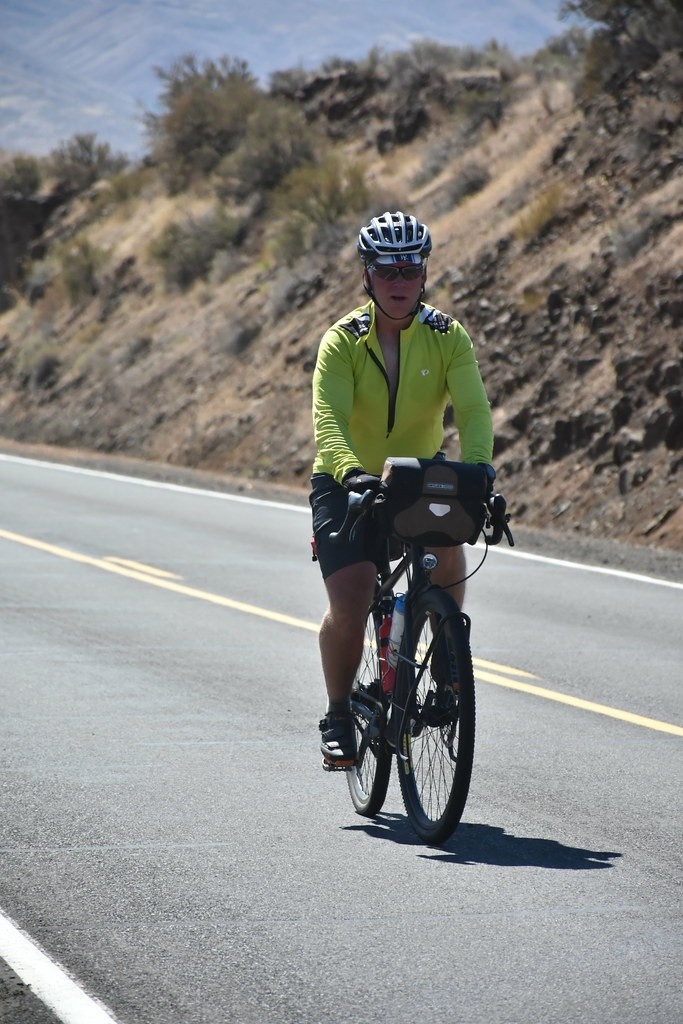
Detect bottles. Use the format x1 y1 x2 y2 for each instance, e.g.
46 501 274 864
385 592 405 665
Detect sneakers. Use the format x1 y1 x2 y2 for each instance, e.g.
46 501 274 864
426 694 459 727
319 710 358 766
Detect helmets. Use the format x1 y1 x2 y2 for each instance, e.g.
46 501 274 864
356 211 432 265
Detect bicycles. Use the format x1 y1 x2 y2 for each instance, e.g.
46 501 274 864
319 453 515 847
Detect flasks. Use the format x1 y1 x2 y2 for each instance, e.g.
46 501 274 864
378 613 396 695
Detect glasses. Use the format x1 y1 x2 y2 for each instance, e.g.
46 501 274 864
366 263 427 281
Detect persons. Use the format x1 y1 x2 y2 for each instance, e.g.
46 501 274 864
308 212 494 766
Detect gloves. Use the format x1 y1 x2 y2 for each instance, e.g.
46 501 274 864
342 472 383 496
